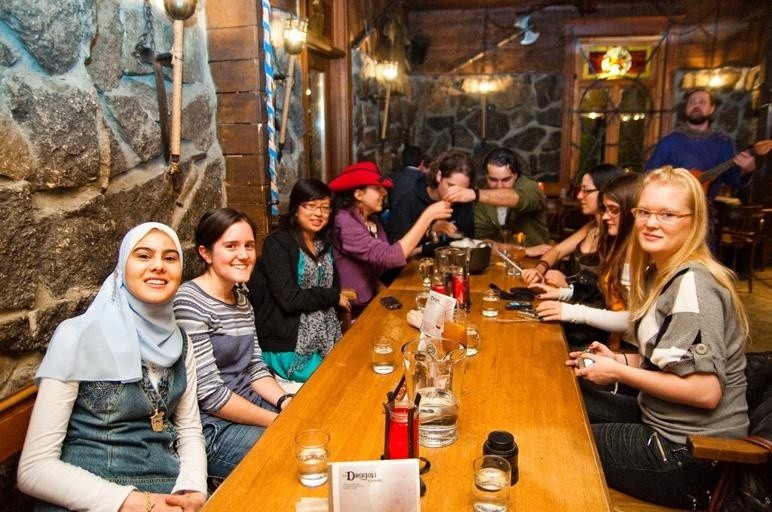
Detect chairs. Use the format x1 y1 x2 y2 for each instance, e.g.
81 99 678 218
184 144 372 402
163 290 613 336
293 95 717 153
1 382 47 512
593 347 772 511
717 203 765 293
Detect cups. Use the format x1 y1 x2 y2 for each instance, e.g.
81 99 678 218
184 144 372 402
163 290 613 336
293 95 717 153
465 322 480 357
387 403 419 458
295 430 329 486
431 273 451 295
416 292 429 309
452 275 470 308
472 455 512 512
435 246 466 298
372 336 395 373
442 308 467 354
482 289 501 317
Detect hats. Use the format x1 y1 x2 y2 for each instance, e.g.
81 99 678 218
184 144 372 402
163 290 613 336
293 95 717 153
328 161 394 193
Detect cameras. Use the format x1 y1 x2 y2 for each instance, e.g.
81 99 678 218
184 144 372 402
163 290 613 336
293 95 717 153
578 349 594 368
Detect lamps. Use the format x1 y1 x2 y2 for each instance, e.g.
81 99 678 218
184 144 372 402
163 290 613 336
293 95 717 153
462 75 513 140
164 0 196 164
379 53 398 140
275 14 309 146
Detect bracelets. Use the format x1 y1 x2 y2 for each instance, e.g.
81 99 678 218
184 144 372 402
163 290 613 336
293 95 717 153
277 393 295 412
536 260 549 269
473 187 480 202
145 491 155 512
622 353 629 366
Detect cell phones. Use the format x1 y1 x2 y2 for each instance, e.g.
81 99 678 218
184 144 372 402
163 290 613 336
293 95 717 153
505 301 532 310
380 297 402 310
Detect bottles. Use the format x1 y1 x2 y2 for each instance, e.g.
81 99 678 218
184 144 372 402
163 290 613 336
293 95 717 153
483 430 519 485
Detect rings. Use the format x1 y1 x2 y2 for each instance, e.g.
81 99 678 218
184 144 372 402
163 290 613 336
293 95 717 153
444 204 447 209
346 297 348 300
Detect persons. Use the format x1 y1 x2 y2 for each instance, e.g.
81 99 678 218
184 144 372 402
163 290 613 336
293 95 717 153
248 179 352 383
527 171 642 355
328 162 453 328
442 147 552 258
386 150 474 258
643 89 756 258
173 209 293 479
564 166 750 509
520 163 622 291
422 155 434 171
389 146 425 207
15 221 208 512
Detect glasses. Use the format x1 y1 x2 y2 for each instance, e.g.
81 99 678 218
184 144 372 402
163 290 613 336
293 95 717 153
598 207 622 215
630 207 694 222
581 188 598 195
301 202 333 213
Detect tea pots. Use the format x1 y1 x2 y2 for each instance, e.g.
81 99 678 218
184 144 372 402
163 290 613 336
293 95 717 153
402 337 467 449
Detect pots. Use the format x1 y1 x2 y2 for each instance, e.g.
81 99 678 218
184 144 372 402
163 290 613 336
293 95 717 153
450 236 492 274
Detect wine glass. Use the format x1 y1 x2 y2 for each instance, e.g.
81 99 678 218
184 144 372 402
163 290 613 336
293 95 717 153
419 257 433 291
498 230 512 272
508 235 527 276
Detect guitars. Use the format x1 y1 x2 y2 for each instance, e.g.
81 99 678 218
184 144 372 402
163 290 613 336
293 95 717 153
688 140 772 196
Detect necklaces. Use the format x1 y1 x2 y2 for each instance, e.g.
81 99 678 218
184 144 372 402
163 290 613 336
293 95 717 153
143 382 169 433
